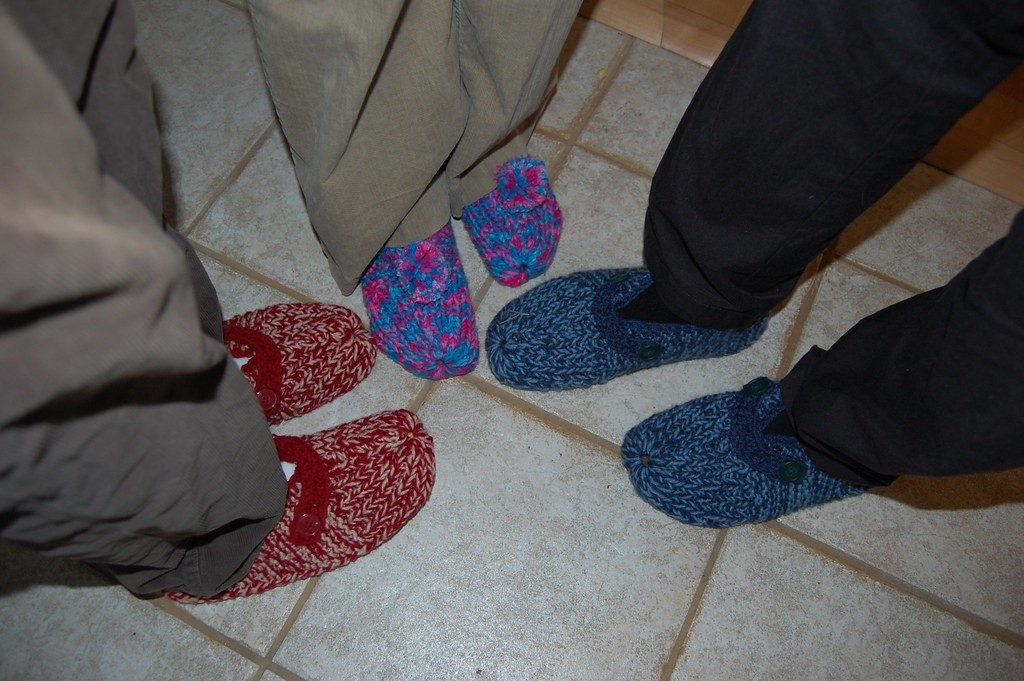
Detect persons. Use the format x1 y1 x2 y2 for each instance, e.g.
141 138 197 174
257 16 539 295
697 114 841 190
242 0 586 382
0 0 439 607
483 0 1023 530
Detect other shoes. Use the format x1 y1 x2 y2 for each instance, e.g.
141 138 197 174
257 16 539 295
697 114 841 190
485 267 769 390
221 302 375 427
161 408 438 604
461 163 563 292
622 376 869 528
360 221 480 380
